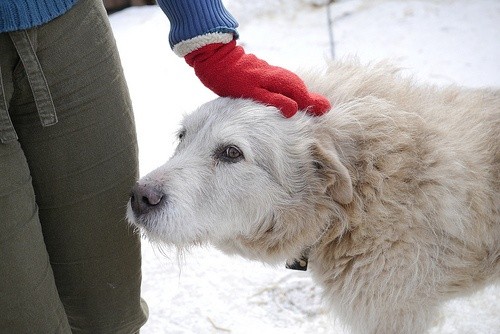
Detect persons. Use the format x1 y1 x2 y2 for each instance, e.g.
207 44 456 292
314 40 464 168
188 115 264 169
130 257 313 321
0 0 334 334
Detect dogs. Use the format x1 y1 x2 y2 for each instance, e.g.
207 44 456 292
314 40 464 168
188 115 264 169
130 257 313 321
125 67 500 334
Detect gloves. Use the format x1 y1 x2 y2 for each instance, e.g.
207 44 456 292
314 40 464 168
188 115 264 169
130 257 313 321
172 31 332 119
